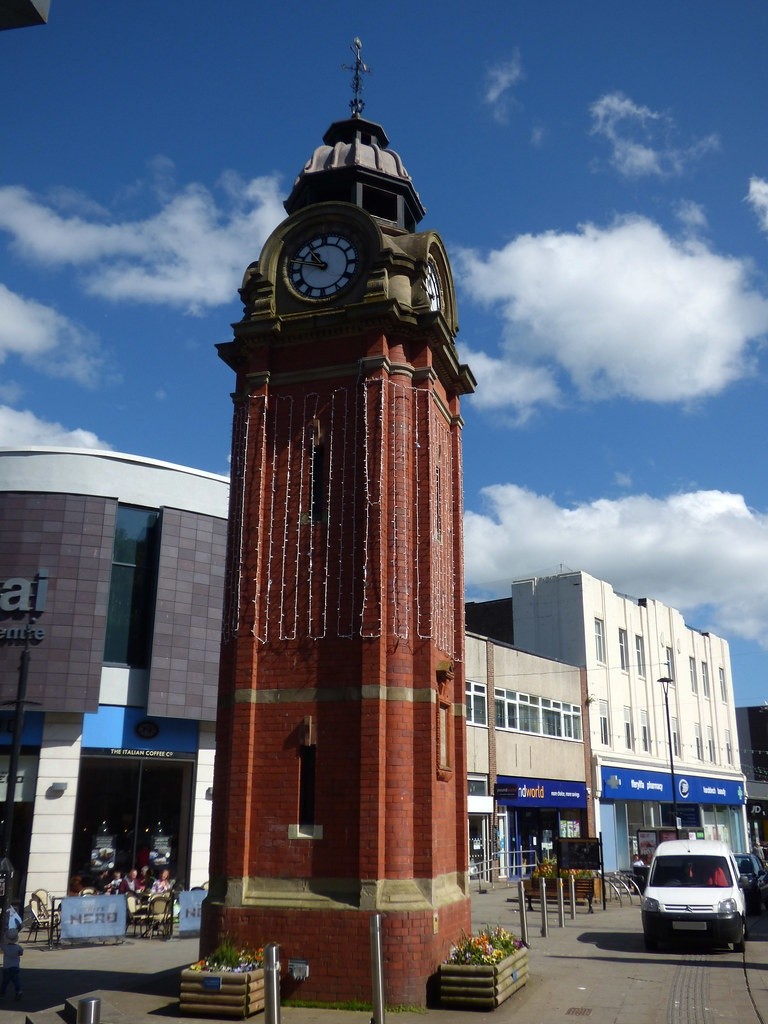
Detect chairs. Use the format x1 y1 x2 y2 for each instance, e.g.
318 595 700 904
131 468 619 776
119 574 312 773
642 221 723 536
26 887 173 945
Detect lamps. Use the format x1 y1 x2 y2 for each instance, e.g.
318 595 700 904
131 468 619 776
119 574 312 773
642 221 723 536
51 781 67 794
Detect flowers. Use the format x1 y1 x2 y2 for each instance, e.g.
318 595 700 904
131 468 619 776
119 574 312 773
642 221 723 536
190 936 264 972
443 921 528 962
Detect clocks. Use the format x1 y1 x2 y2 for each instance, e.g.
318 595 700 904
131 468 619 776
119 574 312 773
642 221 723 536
427 262 441 313
289 233 360 300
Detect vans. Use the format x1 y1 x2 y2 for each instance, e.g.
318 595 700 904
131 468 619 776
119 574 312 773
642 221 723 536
641 839 749 953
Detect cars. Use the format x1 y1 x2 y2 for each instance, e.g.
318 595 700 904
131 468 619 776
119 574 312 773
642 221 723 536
733 853 768 915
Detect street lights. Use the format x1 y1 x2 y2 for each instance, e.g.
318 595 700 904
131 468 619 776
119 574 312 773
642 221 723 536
657 677 679 839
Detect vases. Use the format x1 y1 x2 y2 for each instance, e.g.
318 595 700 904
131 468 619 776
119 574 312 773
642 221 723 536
441 948 529 1010
179 966 282 1016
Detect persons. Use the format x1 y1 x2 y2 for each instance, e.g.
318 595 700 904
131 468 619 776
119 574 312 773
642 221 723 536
632 854 644 867
70 865 173 896
0 928 25 1001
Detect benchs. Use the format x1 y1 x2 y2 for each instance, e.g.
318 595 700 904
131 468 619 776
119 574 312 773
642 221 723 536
522 876 599 913
